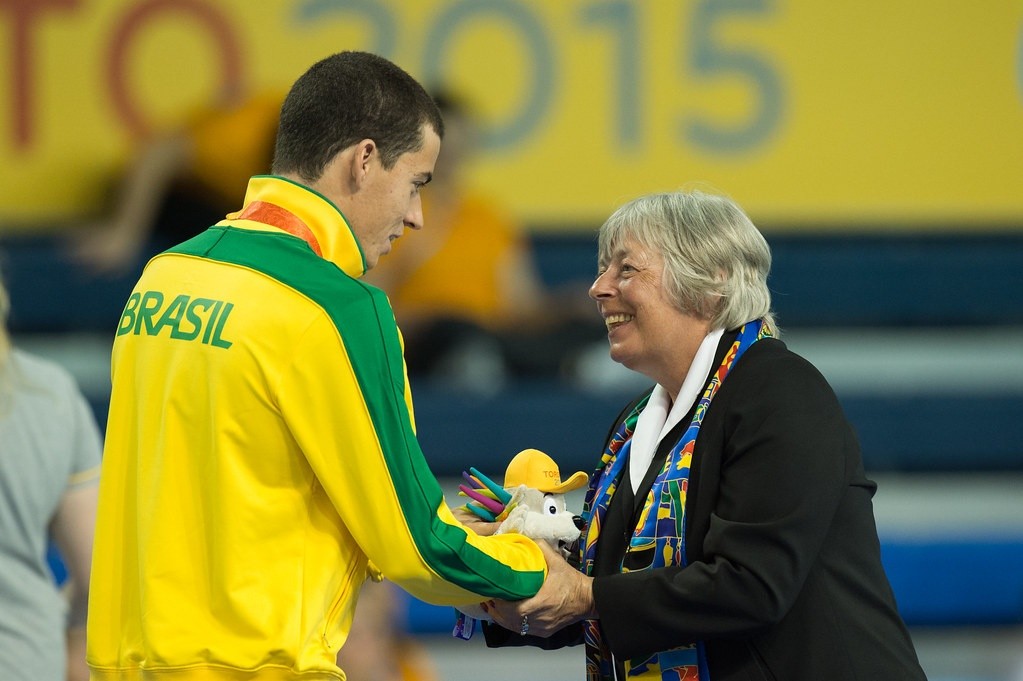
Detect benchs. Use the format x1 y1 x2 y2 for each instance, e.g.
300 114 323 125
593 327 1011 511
0 227 1023 635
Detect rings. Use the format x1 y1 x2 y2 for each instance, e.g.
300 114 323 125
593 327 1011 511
522 615 527 625
519 630 526 635
523 623 529 631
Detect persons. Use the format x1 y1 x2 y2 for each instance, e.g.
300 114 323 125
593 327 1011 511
481 191 927 681
0 51 556 681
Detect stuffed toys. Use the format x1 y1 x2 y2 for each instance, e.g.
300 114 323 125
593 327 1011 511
451 449 590 640
